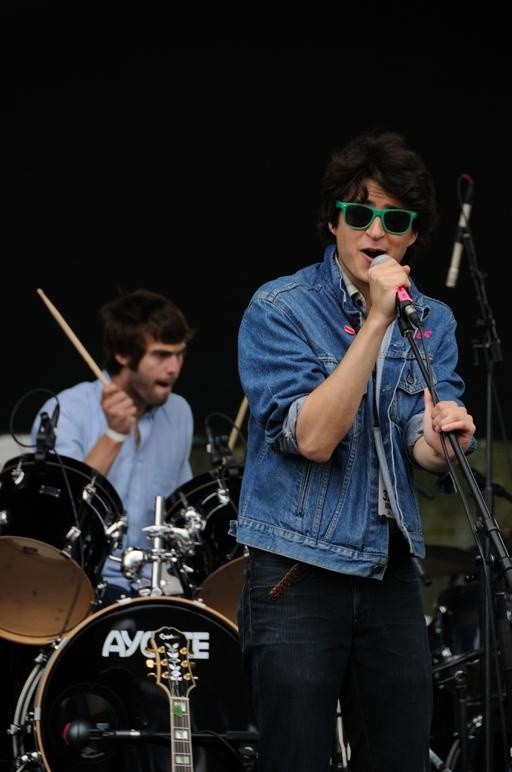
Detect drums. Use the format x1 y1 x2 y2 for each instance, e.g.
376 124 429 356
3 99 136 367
162 464 248 629
8 594 250 772
0 453 128 645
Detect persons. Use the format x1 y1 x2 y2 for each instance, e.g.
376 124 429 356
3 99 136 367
228 133 479 769
29 288 201 602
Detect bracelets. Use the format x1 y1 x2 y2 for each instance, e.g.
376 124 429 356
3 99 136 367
105 426 130 443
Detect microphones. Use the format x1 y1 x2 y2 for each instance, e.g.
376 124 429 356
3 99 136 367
64 721 99 747
369 255 422 328
445 185 475 290
203 424 223 466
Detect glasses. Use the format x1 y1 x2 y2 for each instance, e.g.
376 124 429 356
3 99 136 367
335 200 420 235
